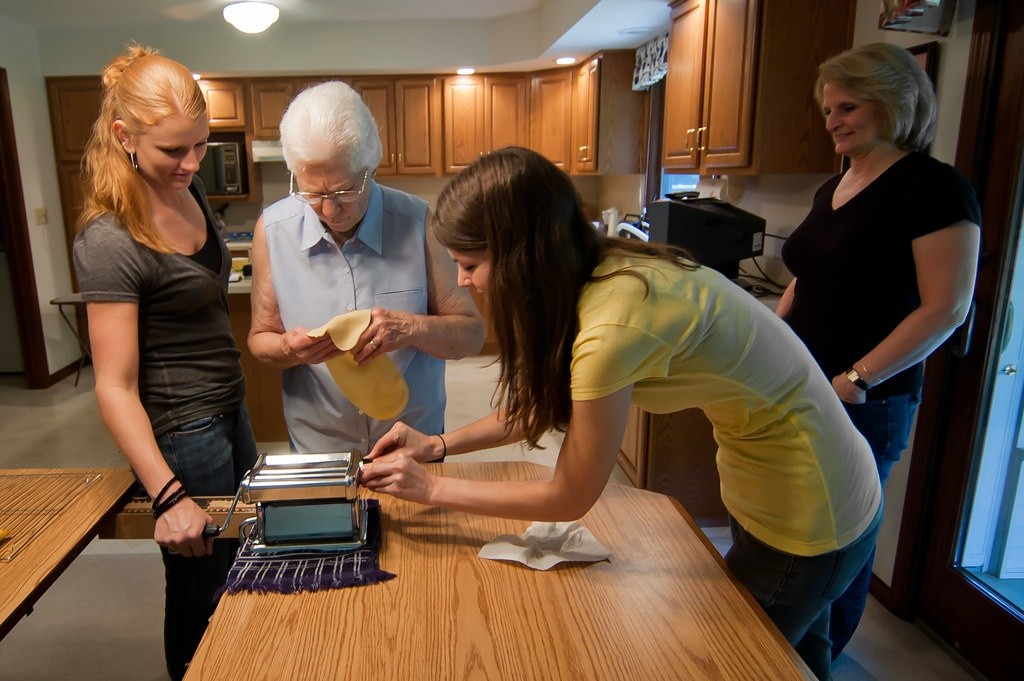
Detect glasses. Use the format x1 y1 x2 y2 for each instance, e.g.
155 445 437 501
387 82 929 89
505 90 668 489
289 166 368 205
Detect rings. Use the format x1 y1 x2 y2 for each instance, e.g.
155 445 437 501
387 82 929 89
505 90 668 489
169 551 178 554
370 341 377 349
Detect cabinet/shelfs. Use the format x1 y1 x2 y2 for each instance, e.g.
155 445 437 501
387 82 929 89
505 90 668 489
44 48 645 358
616 406 727 527
662 0 858 178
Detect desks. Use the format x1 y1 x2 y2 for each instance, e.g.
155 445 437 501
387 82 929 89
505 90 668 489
179 464 825 681
50 295 93 386
0 467 140 644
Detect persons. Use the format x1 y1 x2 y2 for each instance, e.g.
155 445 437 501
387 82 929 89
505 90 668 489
777 42 982 664
72 45 259 681
361 144 884 681
248 82 485 459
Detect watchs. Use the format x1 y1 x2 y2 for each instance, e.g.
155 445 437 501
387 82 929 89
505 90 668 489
846 364 871 391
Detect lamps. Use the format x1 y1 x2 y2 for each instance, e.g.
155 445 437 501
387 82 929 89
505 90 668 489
223 2 280 34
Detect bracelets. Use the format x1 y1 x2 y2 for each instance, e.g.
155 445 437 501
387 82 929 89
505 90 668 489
152 476 190 519
437 434 446 459
860 361 878 383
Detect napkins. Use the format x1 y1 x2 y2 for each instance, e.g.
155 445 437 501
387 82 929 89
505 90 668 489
478 519 614 570
